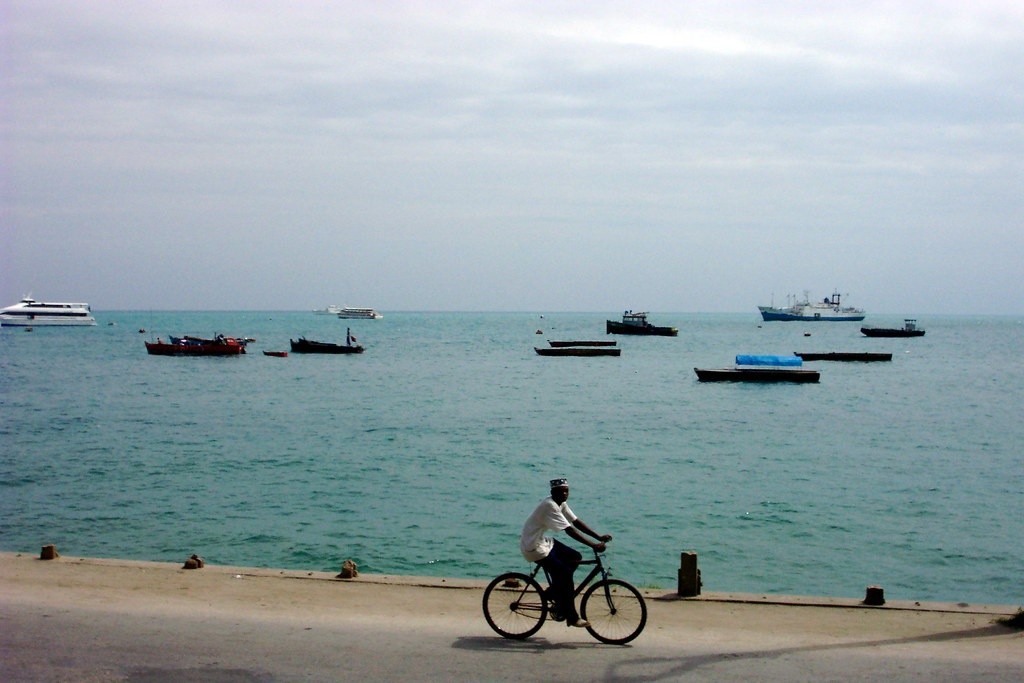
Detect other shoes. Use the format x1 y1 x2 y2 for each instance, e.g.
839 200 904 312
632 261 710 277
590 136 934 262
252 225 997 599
567 618 591 628
545 586 556 601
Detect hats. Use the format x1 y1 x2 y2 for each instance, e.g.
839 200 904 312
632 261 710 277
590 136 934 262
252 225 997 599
550 478 569 488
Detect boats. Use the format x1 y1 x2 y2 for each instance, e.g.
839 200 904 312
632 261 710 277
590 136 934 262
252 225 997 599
25 327 33 332
290 327 364 353
108 323 115 325
337 308 383 319
144 338 243 354
139 329 145 333
794 352 892 362
534 347 621 357
312 305 342 314
607 310 678 335
169 335 246 346
548 341 617 347
694 368 820 383
758 294 865 321
0 299 97 325
861 320 925 336
263 351 287 357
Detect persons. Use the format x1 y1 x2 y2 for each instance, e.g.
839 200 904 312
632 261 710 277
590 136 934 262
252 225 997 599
519 477 613 627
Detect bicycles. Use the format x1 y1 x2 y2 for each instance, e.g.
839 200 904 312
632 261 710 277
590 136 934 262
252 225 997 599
483 542 647 644
